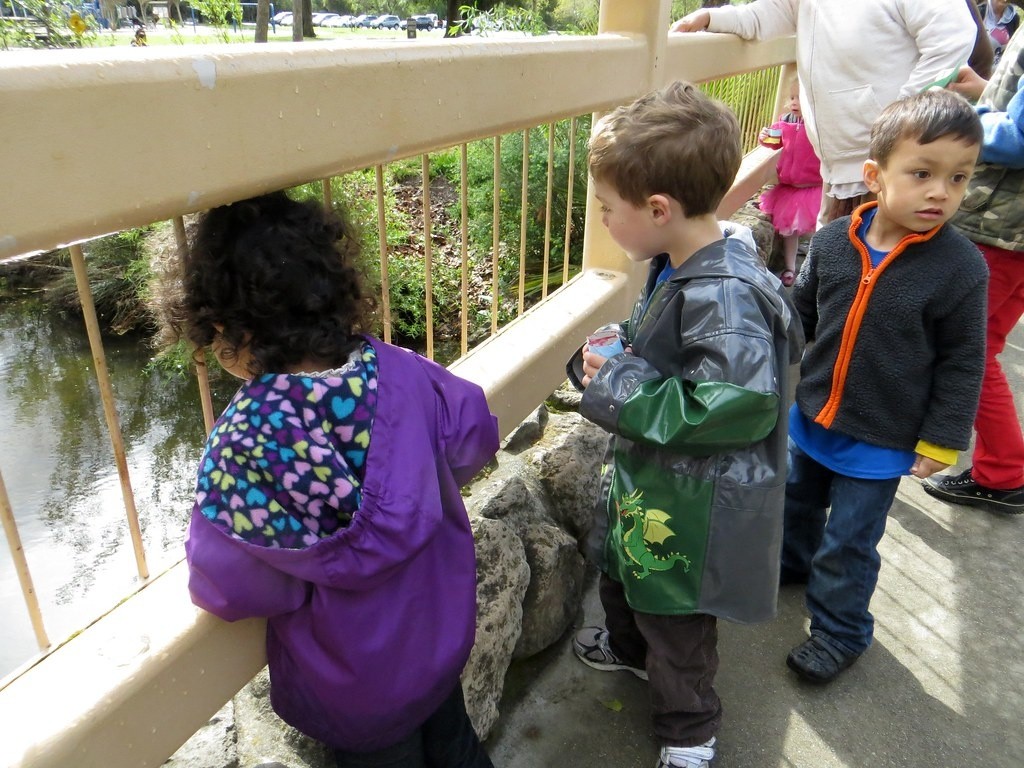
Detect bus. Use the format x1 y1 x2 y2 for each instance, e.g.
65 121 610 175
428 14 438 29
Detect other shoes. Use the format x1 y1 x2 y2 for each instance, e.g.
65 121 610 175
780 270 797 286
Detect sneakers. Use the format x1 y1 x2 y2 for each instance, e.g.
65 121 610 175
655 736 717 767
784 634 858 684
921 467 1024 515
571 625 649 682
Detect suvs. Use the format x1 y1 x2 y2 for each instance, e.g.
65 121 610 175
398 15 434 31
369 14 400 30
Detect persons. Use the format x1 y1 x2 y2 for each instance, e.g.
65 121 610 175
131 29 148 47
782 94 987 684
758 77 822 285
669 0 977 231
921 22 1024 514
571 89 806 768
169 190 498 768
974 0 1024 69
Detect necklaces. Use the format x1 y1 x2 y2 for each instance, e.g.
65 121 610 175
795 115 806 131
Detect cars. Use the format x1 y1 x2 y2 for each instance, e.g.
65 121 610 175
268 11 378 28
